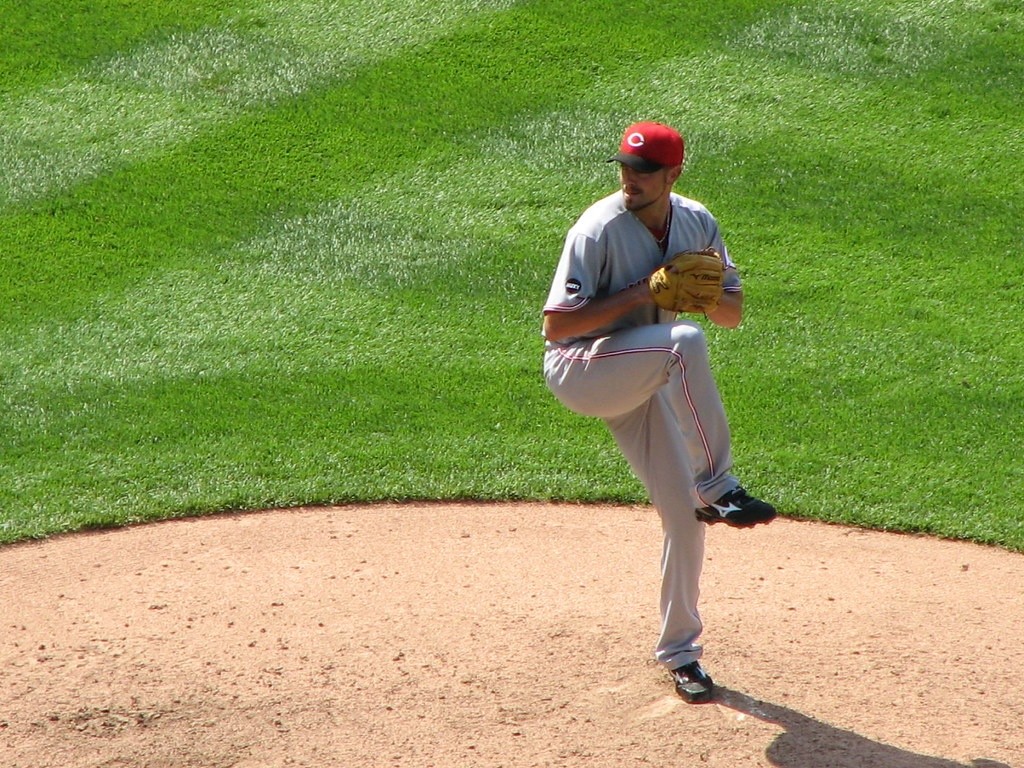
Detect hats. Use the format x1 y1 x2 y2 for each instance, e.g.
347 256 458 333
607 122 684 173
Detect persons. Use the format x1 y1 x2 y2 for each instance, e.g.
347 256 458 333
543 122 778 703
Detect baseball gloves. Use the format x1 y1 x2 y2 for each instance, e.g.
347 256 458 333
648 246 725 314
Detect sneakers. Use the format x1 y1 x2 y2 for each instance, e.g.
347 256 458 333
695 485 777 529
668 659 713 703
